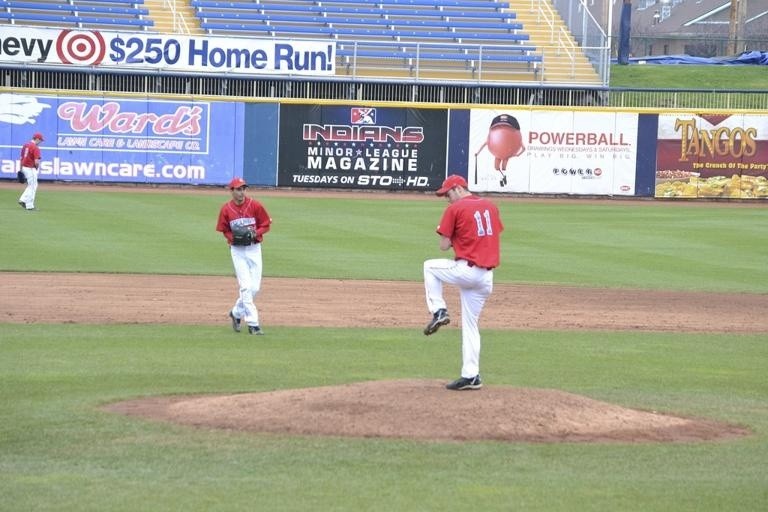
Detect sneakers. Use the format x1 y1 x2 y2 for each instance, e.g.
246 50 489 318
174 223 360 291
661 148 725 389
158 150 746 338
446 374 482 392
18 199 38 211
229 310 242 333
500 175 507 187
424 311 451 336
248 324 263 335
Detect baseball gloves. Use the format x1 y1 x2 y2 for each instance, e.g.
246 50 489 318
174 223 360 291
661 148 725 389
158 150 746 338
18 171 27 183
232 226 252 246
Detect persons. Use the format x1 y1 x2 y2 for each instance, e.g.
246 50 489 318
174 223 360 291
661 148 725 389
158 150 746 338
424 175 503 389
216 178 272 335
18 133 44 211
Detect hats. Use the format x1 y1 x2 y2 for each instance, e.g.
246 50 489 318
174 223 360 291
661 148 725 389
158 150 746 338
228 177 248 190
434 174 469 197
489 113 520 130
32 133 45 142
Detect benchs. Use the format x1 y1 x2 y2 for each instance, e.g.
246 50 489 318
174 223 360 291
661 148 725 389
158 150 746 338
188 0 546 83
1 1 158 37
